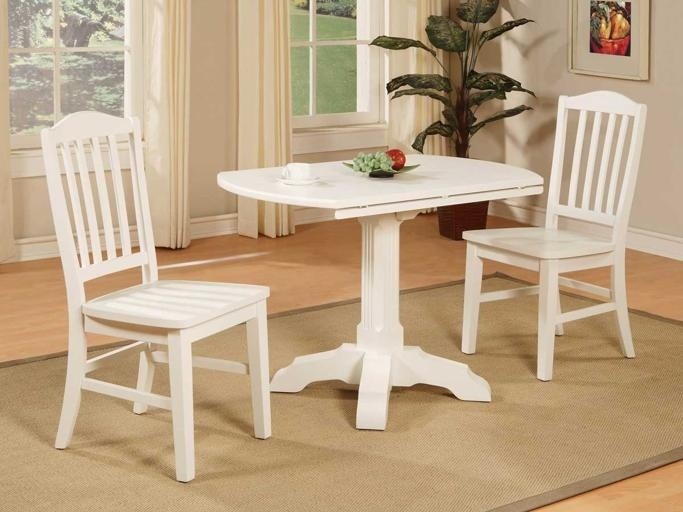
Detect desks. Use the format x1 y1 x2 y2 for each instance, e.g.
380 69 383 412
210 148 546 433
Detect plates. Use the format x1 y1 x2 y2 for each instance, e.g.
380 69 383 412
276 173 322 186
339 161 420 179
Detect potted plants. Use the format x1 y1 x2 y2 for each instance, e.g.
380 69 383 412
366 1 543 243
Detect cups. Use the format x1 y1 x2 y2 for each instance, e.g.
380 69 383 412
280 162 310 182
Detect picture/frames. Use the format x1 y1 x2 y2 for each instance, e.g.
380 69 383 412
563 1 653 81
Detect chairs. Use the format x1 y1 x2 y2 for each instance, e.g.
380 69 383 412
454 86 649 383
35 107 276 485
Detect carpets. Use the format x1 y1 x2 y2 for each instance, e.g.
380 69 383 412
2 267 681 511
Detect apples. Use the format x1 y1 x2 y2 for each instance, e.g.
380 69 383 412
386 149 406 171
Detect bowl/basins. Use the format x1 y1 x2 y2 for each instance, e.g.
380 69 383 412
590 35 629 55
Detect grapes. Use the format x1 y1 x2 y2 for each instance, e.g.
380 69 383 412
352 151 392 172
